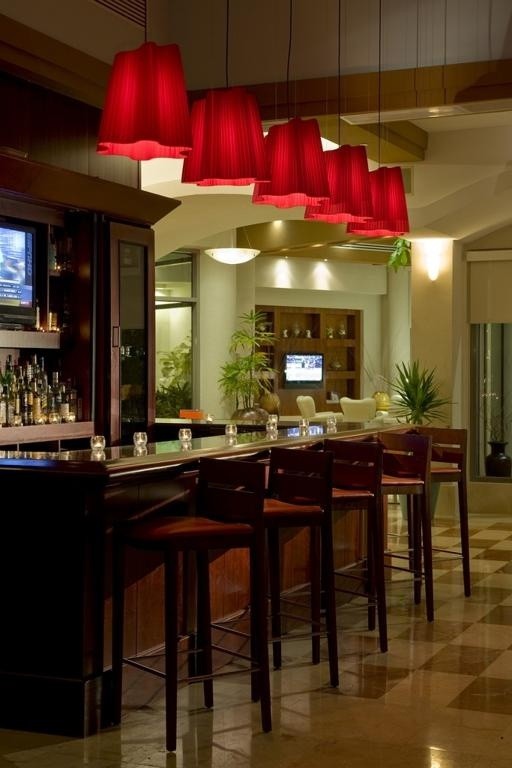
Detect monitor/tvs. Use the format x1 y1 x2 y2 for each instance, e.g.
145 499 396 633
0 216 39 329
281 353 325 389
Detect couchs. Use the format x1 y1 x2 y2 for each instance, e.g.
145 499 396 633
340 396 388 427
296 395 344 422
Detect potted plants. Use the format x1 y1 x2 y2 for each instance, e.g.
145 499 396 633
220 309 275 421
379 360 454 522
482 412 511 479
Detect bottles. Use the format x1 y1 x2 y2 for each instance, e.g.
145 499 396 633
0 353 83 425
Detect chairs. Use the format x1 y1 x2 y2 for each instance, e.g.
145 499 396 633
185 446 340 687
382 425 472 604
270 439 389 671
107 456 275 751
363 433 434 630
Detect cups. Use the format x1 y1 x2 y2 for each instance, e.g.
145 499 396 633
178 427 192 442
90 435 106 450
91 450 106 461
224 423 238 435
265 419 278 432
298 417 310 429
10 412 77 425
134 446 148 456
132 431 148 445
327 425 338 434
180 442 192 452
299 428 310 438
268 414 278 422
266 432 278 441
326 415 338 427
225 435 238 448
205 413 215 423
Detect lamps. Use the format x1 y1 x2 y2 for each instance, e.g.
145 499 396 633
180 0 270 190
92 0 193 163
250 0 330 211
302 0 375 229
347 0 412 242
205 226 261 264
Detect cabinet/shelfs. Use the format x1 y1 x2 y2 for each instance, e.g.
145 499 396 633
255 304 366 414
0 201 95 452
97 218 156 444
